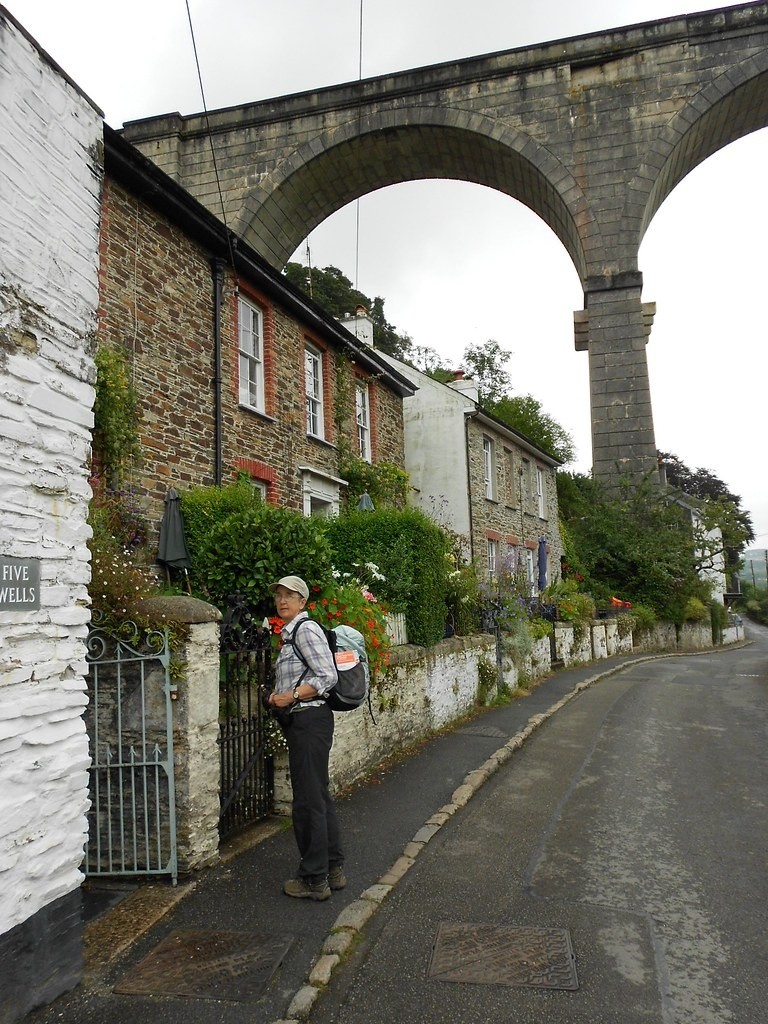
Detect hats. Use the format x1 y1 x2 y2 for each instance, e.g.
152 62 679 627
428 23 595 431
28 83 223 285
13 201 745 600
269 575 309 601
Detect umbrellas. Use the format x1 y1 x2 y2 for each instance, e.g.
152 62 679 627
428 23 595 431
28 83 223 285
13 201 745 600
359 492 376 513
537 535 548 591
160 487 194 600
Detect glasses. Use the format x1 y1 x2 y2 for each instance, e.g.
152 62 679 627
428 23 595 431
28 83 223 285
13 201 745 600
274 594 301 602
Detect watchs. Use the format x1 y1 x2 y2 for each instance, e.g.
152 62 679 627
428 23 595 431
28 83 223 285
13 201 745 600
291 686 301 702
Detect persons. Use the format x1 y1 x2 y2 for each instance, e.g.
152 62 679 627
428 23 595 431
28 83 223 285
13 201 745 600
270 576 347 901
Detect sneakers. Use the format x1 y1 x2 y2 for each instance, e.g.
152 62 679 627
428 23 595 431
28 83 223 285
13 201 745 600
328 865 347 889
284 876 331 900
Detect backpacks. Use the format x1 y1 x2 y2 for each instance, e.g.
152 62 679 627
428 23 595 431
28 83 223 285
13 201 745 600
292 617 366 711
